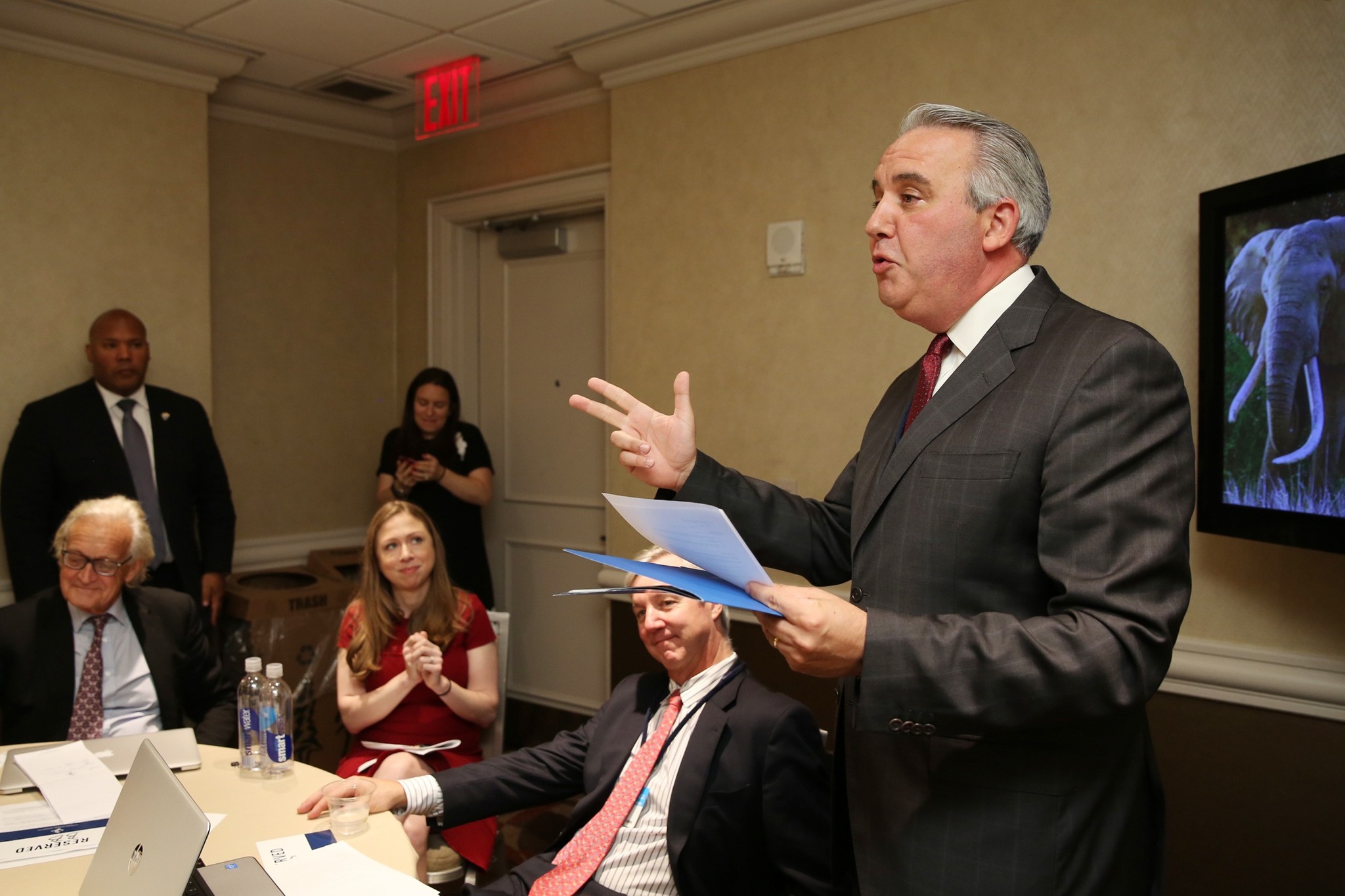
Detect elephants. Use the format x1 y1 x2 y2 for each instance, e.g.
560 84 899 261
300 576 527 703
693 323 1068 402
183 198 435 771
1224 217 1345 495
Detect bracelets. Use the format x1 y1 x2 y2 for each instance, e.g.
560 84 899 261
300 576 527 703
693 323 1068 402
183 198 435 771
391 484 408 499
437 468 446 482
438 680 451 696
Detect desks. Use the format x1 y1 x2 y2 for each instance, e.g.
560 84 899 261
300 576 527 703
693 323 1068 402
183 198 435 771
0 741 420 896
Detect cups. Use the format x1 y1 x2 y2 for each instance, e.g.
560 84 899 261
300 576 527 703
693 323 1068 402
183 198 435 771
322 778 377 838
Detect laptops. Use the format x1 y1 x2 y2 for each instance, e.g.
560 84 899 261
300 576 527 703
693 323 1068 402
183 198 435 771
75 738 285 896
0 727 203 795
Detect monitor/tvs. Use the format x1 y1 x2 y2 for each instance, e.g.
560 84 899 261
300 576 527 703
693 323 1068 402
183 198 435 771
1196 153 1345 556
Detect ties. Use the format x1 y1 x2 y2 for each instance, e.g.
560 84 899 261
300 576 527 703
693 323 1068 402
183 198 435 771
904 334 955 435
67 613 112 743
527 687 683 896
117 398 168 572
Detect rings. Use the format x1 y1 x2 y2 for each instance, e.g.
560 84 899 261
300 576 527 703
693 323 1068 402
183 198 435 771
773 638 779 647
430 656 433 663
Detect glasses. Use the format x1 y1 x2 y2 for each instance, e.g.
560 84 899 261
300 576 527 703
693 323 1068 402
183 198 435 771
60 539 134 577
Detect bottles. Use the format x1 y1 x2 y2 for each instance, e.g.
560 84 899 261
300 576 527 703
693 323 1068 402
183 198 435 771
237 657 267 771
259 663 294 774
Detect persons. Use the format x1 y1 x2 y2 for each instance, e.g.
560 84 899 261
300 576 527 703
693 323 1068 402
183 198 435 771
297 545 832 896
336 500 499 885
0 495 239 748
0 308 236 643
376 368 496 610
569 102 1196 896
